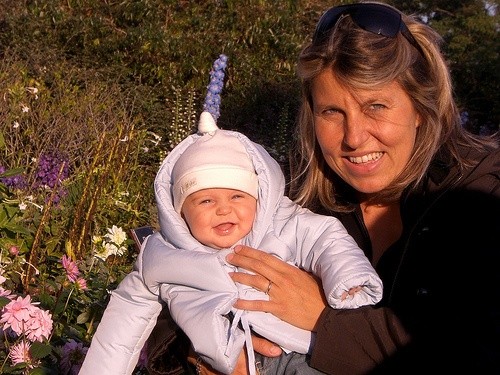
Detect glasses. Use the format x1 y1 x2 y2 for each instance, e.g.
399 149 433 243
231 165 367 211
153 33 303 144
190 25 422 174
312 3 424 55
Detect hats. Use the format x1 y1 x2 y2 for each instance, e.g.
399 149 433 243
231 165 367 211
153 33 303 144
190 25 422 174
172 132 260 217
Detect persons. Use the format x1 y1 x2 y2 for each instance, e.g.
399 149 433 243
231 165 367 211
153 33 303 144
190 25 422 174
76 112 384 375
235 0 498 374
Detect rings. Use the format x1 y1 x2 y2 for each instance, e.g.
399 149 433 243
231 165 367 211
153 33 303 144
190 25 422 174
265 280 272 295
